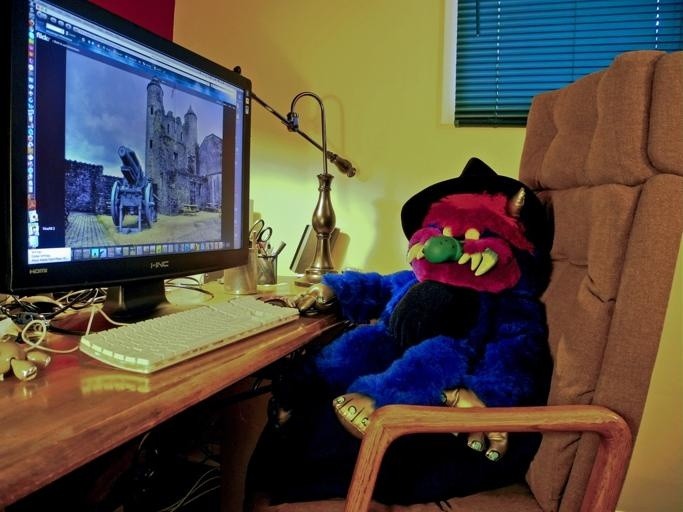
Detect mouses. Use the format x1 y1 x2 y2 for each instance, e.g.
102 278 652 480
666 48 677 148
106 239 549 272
257 296 297 308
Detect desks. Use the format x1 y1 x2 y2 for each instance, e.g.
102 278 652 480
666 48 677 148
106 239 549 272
0 274 350 512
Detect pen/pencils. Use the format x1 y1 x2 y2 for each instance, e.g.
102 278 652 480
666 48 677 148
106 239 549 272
252 231 257 290
243 264 253 290
256 241 286 284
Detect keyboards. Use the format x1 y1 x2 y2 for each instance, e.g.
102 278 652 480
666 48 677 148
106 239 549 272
80 297 300 375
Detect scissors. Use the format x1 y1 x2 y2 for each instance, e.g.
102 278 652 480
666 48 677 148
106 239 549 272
248 219 273 244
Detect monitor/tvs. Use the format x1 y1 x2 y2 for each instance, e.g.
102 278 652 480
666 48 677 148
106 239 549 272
0 0 251 333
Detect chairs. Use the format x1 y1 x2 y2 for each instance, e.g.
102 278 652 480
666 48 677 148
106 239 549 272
251 49 683 512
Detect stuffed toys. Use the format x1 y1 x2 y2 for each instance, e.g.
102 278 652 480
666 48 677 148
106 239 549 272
242 157 554 512
